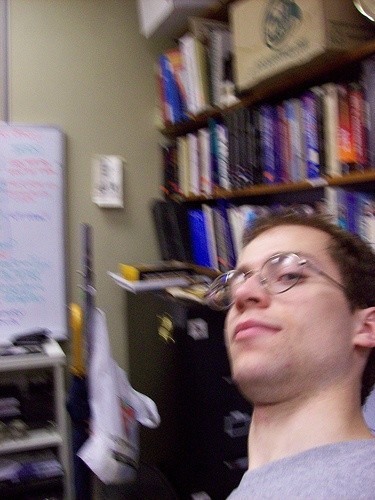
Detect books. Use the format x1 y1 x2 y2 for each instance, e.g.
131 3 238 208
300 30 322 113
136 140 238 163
150 16 241 126
148 82 375 197
107 186 375 293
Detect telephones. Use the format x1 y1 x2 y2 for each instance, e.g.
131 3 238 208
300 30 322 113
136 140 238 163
92 155 124 208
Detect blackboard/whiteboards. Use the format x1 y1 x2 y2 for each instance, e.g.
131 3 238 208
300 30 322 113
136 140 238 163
0 126 70 345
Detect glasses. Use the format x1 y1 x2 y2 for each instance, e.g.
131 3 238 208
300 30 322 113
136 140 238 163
203 253 352 309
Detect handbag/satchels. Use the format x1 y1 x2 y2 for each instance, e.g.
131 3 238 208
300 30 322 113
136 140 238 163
75 303 159 487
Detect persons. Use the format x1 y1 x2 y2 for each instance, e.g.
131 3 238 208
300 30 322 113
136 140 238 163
201 215 375 499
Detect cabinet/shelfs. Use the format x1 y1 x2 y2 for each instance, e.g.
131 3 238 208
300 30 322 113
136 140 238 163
0 340 77 500
156 1 374 280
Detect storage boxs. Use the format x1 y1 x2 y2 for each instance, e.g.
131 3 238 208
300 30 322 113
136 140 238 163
230 0 375 98
139 1 220 40
